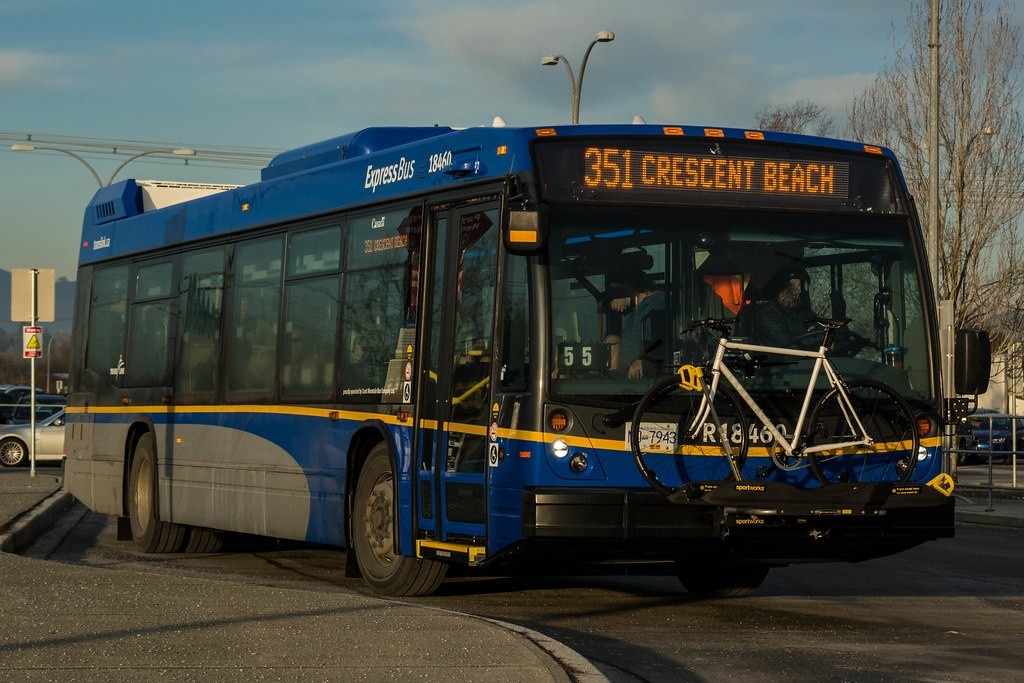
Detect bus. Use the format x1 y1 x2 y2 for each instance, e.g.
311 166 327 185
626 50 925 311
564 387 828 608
65 123 951 601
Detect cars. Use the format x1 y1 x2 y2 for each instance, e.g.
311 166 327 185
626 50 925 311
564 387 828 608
968 413 1024 465
0 408 66 467
0 382 67 425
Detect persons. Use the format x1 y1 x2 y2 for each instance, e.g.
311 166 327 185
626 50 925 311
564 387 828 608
598 243 665 381
744 267 851 358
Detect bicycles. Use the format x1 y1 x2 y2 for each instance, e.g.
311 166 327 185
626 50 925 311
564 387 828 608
629 319 917 508
